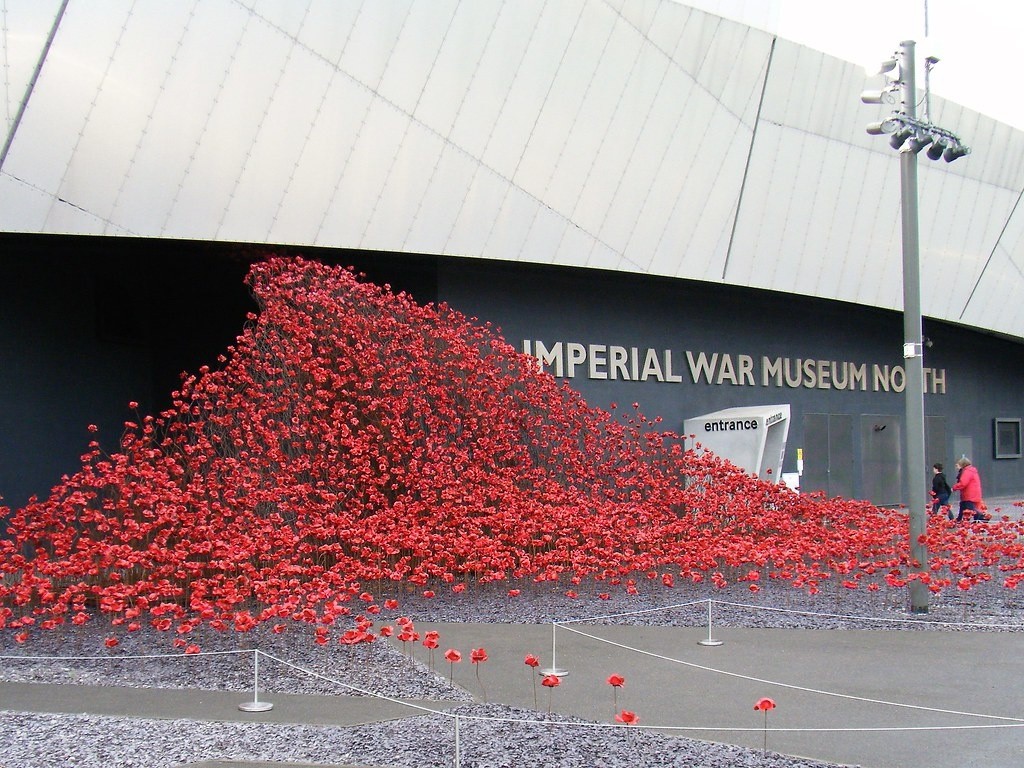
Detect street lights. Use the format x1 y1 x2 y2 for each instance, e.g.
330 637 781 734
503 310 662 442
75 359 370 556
857 39 971 616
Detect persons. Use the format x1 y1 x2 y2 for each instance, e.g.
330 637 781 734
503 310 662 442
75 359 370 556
950 457 990 523
929 463 955 525
